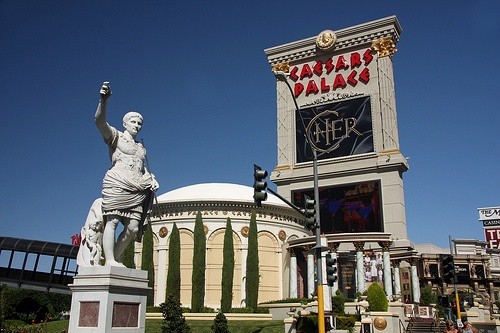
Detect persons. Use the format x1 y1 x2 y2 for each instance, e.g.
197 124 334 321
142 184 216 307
443 320 459 333
94 80 161 268
459 316 479 333
86 221 105 262
